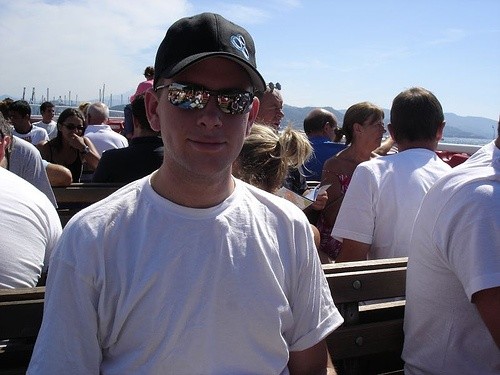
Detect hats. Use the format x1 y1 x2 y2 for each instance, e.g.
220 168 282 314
152 13 266 86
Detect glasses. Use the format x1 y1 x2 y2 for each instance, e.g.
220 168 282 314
58 123 86 130
151 82 259 116
266 82 281 90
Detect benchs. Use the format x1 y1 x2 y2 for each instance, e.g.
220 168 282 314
1 258 407 373
50 180 320 228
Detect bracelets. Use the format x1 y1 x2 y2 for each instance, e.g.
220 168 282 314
81 145 89 156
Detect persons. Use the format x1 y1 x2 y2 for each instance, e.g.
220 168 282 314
231 124 320 265
322 102 387 260
0 112 59 209
27 10 344 375
0 97 14 121
37 108 101 183
330 86 452 299
32 101 58 140
123 66 155 139
0 118 62 290
83 101 129 156
400 118 500 375
92 94 164 182
300 108 395 187
252 82 328 212
8 99 50 147
